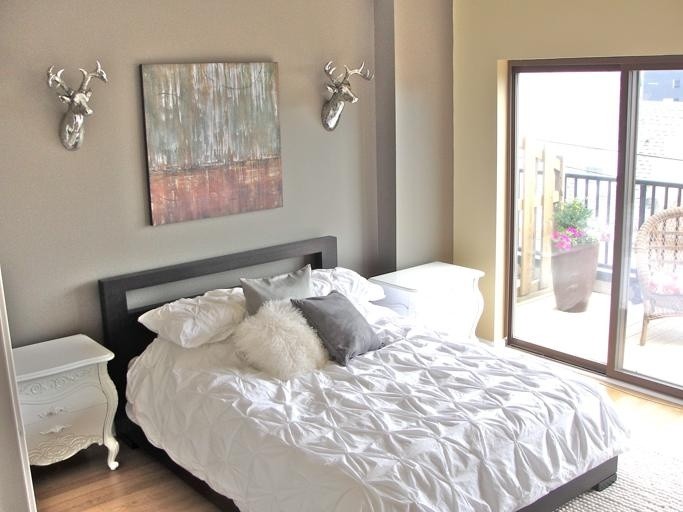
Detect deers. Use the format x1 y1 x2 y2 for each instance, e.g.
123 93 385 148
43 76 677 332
321 59 375 132
47 59 108 152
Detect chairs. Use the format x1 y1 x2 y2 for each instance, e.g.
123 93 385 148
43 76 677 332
634 206 683 347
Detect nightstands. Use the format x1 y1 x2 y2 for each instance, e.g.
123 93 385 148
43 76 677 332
9 333 121 473
367 259 486 343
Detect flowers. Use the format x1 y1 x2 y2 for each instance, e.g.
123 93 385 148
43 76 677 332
549 196 596 257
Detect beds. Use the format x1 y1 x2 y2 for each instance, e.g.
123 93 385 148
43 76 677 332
94 234 633 512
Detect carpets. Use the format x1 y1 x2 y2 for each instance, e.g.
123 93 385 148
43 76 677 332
553 471 683 512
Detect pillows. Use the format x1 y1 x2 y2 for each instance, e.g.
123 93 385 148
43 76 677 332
289 288 383 368
136 285 245 351
311 267 386 304
237 262 313 317
229 299 331 386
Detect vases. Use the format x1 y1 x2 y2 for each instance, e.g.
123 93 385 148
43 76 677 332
550 242 598 313
